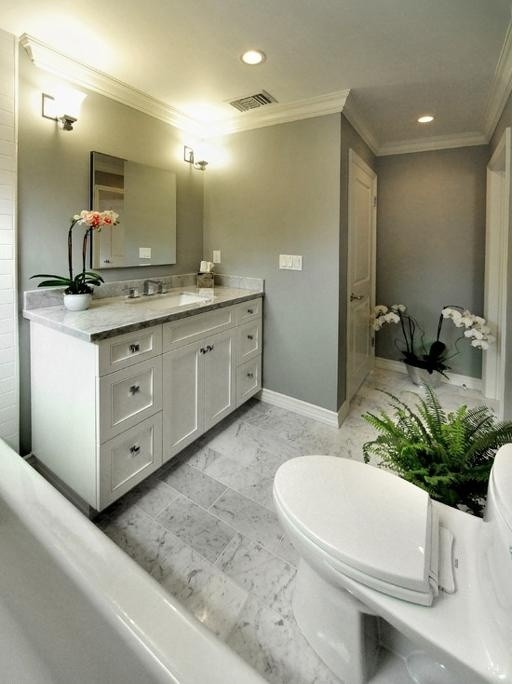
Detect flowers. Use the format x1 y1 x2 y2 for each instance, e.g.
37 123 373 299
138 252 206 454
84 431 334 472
92 208 119 234
29 209 104 292
367 303 497 380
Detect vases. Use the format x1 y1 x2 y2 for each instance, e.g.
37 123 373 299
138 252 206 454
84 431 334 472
64 294 92 312
403 355 442 389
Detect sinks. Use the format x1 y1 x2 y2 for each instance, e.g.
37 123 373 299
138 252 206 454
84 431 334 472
123 290 212 311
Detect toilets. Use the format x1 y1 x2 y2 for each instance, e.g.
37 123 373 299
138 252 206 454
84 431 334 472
272 443 511 683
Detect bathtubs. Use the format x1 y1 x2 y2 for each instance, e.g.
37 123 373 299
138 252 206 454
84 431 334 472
0 438 271 683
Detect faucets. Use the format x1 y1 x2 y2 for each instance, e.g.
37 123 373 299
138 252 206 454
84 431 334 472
143 278 163 295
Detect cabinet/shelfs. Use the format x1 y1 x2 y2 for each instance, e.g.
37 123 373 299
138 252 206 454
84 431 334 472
30 295 263 513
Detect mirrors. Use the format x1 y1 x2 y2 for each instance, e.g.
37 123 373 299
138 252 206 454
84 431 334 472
88 150 178 270
